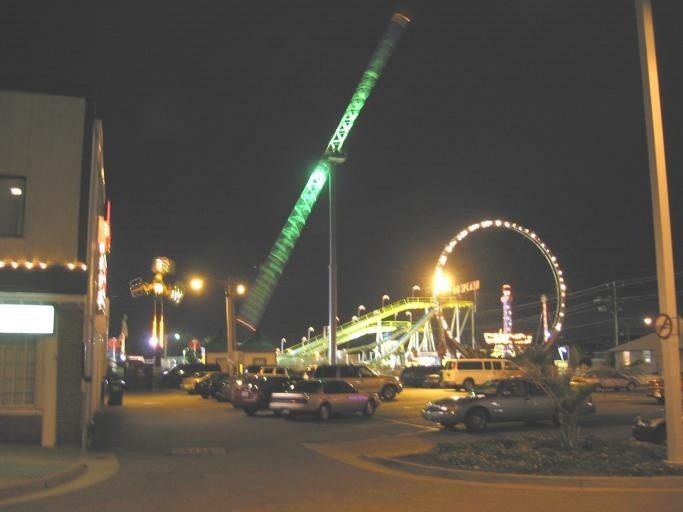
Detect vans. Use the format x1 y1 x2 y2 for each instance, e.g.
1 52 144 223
162 361 223 388
442 357 528 393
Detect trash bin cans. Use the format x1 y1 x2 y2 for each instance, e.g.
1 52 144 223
107 380 125 405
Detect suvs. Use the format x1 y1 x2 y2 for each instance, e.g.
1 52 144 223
301 362 405 403
244 364 295 379
400 367 432 387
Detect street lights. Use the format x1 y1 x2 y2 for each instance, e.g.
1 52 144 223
322 147 349 365
186 272 247 378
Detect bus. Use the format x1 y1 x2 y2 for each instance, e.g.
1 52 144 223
269 377 380 420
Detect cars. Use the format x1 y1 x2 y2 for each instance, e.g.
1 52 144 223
107 367 129 406
644 373 683 408
421 377 599 429
569 368 644 393
423 373 453 388
228 373 292 417
177 369 242 404
